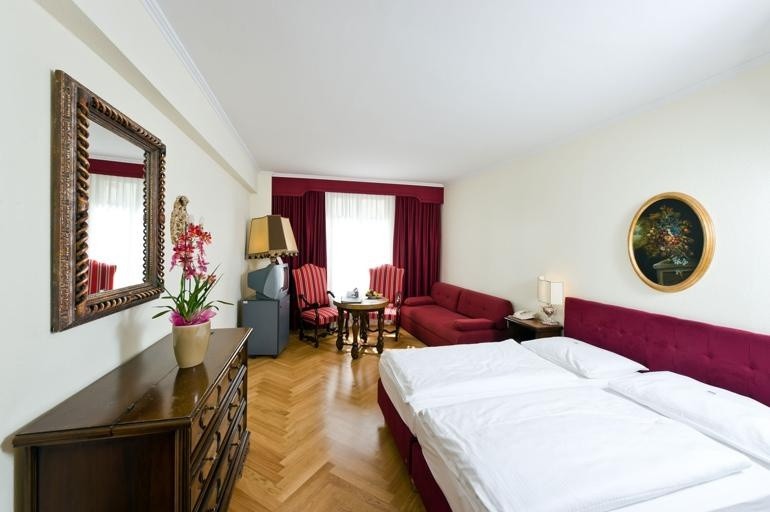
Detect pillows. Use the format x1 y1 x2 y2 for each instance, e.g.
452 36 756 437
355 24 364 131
610 361 770 465
519 333 651 382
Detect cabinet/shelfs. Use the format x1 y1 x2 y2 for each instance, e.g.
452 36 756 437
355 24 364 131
7 327 261 512
237 292 294 359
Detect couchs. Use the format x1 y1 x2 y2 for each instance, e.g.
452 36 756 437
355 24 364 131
398 279 513 348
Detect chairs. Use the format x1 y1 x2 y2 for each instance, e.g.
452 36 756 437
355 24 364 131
292 262 351 349
363 263 408 343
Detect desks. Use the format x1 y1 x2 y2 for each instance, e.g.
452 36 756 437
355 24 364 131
331 297 391 360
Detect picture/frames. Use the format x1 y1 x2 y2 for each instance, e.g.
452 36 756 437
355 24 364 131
624 189 718 295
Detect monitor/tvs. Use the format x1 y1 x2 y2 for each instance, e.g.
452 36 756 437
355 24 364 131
247 263 290 301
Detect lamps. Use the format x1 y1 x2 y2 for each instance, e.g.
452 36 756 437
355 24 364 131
536 275 567 326
244 213 301 266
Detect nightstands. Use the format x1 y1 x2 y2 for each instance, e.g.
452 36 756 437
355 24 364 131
503 313 565 345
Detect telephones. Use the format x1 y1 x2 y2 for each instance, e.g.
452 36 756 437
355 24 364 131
513 310 537 319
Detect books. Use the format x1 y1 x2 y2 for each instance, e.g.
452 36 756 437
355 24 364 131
341 295 361 304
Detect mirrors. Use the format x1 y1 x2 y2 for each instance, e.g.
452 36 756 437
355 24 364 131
50 68 166 334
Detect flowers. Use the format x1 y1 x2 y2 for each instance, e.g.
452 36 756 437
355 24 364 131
632 202 697 267
149 221 236 327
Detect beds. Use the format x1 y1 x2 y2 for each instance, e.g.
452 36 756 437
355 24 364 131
412 334 770 511
372 295 657 477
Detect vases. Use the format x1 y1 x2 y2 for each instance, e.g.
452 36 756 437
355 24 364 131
169 319 213 369
652 254 700 287
